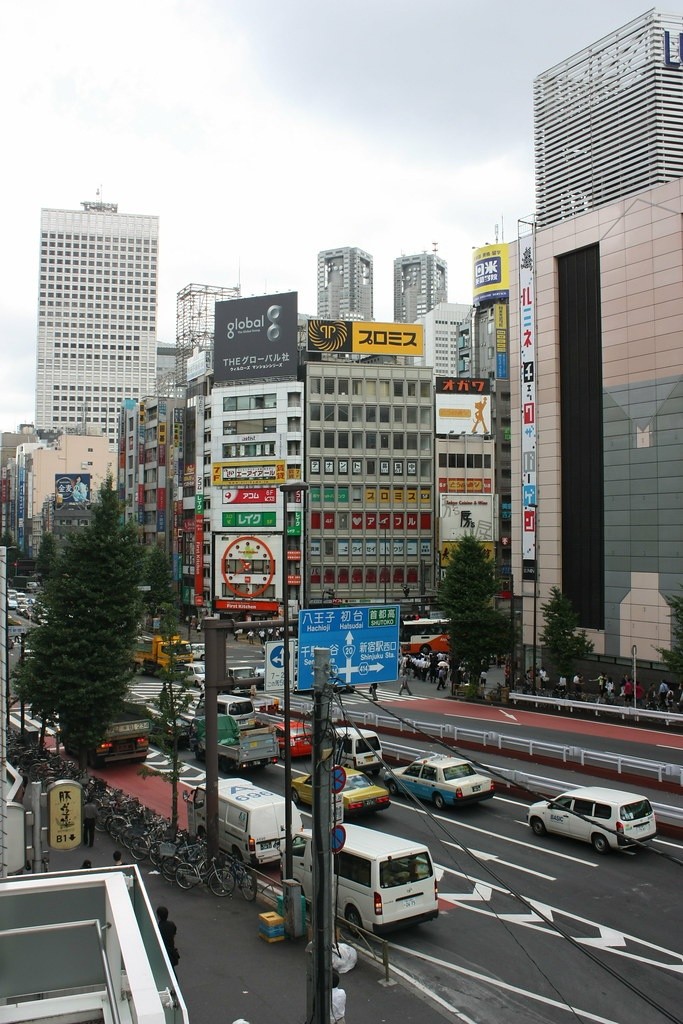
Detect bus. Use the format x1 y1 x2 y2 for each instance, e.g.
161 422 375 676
400 617 453 655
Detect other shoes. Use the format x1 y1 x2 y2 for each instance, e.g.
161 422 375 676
443 686 446 689
372 699 378 701
437 689 440 691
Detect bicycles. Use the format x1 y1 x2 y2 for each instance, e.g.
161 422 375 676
6 727 255 901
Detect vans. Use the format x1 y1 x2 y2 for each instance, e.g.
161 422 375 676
278 823 441 934
334 728 385 776
194 692 256 730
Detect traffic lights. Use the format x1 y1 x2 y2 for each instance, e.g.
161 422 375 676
328 589 334 598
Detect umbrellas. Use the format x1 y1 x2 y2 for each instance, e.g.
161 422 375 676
437 661 449 669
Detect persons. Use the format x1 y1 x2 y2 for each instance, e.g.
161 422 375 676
369 683 377 701
398 651 449 695
156 906 180 984
481 653 683 727
81 860 91 868
113 850 128 866
330 972 346 1024
18 769 28 797
83 797 99 847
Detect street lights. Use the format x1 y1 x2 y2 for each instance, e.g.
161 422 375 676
528 503 537 692
379 523 387 604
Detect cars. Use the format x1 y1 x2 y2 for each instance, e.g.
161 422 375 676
293 768 392 818
385 753 496 809
7 589 50 625
528 786 659 853
9 639 15 649
181 642 264 690
270 722 314 761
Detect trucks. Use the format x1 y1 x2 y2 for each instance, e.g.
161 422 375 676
131 636 194 678
57 703 154 768
184 778 303 866
13 576 39 592
188 714 281 775
